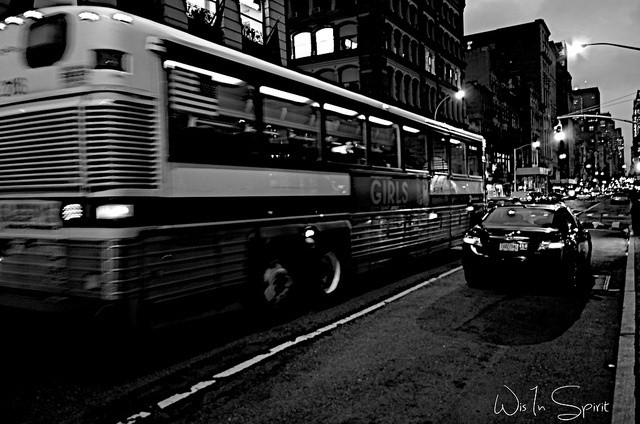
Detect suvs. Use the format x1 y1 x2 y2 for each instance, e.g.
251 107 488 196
526 192 541 203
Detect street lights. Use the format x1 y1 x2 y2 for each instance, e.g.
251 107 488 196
576 41 640 54
514 141 539 193
434 91 464 121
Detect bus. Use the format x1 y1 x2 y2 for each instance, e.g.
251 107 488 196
0 6 486 321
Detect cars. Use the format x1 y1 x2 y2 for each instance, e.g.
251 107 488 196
486 197 525 211
611 192 629 205
604 191 612 197
461 203 592 288
591 191 600 197
